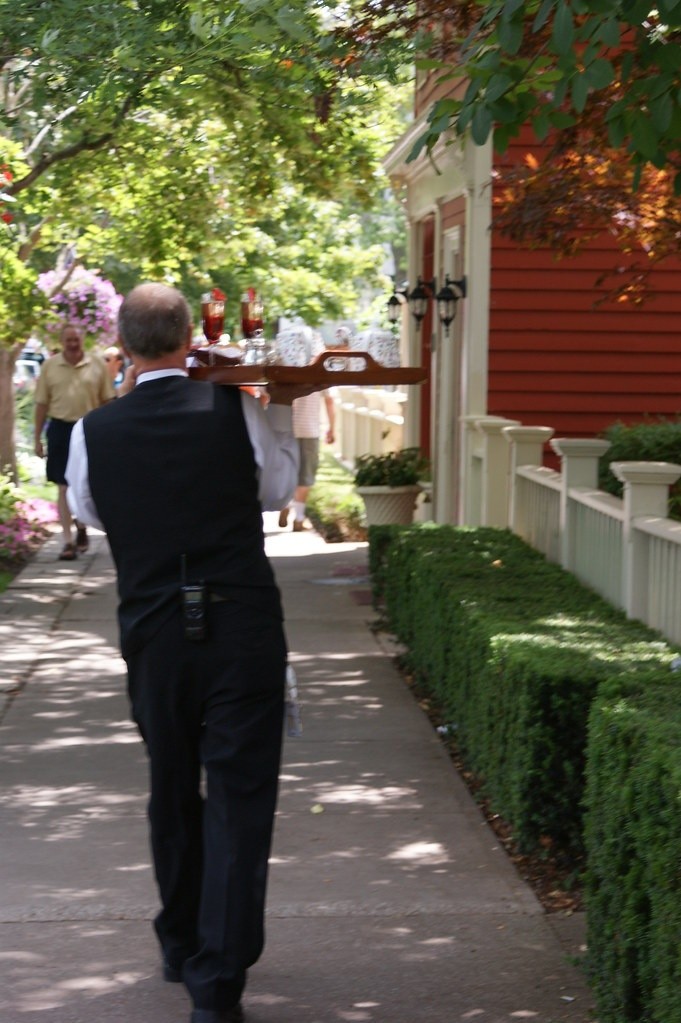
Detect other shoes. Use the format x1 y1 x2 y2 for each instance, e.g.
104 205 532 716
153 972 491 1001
279 508 289 527
192 1003 243 1023
293 520 303 530
164 954 186 983
60 544 75 560
75 518 88 551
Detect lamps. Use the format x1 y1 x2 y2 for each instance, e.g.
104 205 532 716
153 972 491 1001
435 273 467 338
387 288 408 325
410 276 435 330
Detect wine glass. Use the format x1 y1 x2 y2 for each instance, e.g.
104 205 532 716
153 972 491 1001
241 293 263 365
200 301 225 367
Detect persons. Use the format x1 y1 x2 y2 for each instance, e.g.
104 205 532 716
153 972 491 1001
102 345 124 390
63 284 302 1023
33 324 115 562
258 354 336 533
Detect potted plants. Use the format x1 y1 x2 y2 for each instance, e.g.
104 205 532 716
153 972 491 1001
351 445 428 528
